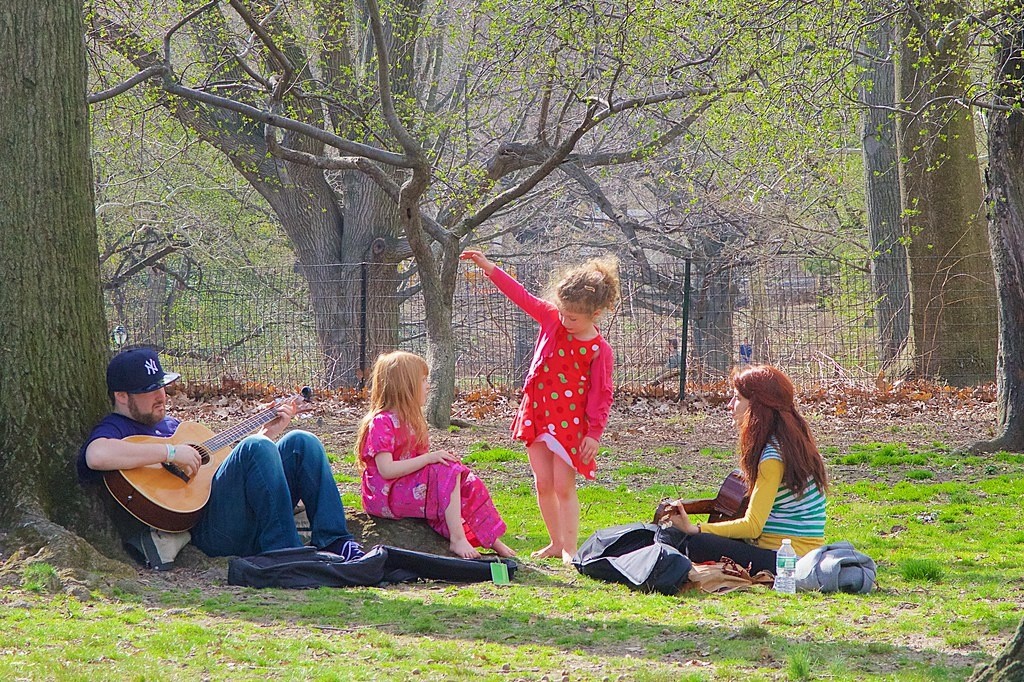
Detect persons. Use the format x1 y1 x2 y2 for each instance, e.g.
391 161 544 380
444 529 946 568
666 339 681 370
740 337 752 362
658 365 826 575
460 250 615 559
76 349 363 562
357 350 516 558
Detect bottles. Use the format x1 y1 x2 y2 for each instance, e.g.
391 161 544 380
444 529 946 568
776 538 797 594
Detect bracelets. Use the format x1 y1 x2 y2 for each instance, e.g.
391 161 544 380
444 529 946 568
167 444 174 462
697 523 701 534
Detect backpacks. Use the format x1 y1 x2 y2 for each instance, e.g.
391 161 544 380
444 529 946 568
571 519 692 596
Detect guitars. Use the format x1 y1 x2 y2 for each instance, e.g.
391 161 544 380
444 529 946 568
101 391 314 535
651 466 754 531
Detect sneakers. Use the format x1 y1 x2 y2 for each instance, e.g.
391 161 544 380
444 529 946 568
321 536 366 561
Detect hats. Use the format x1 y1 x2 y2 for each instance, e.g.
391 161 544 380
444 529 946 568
107 348 181 395
